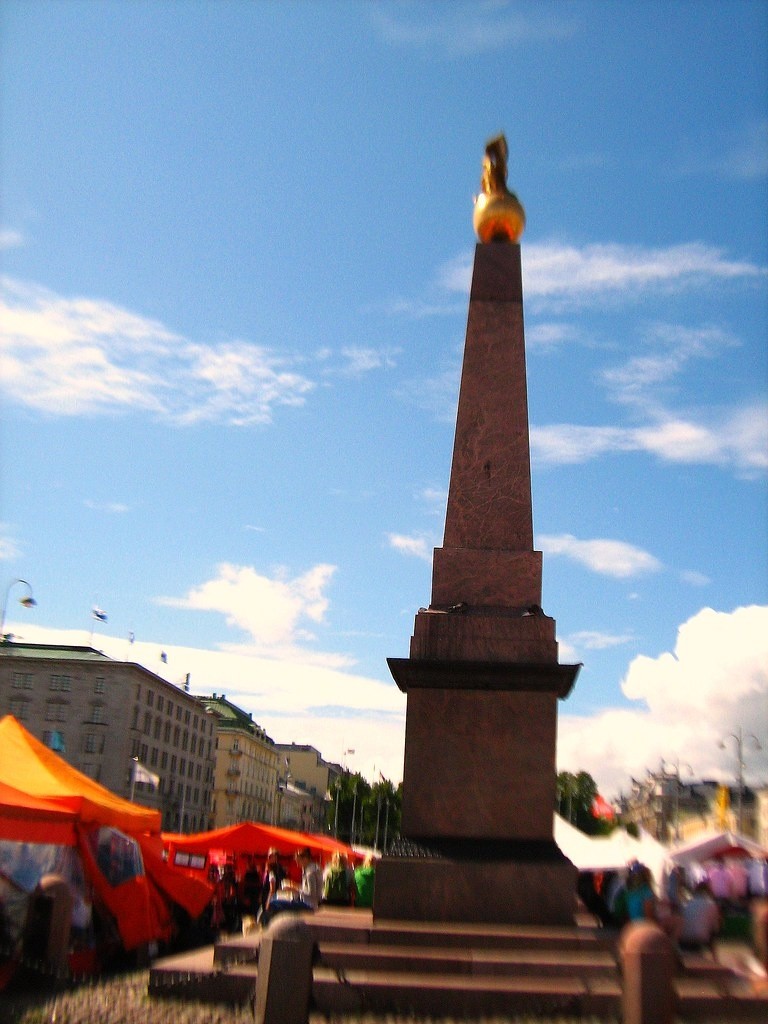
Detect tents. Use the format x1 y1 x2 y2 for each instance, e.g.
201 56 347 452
0 711 367 948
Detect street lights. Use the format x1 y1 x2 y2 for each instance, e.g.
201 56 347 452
718 731 762 839
0 577 37 644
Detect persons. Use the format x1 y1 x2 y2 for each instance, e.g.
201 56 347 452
354 853 379 906
322 852 353 905
262 848 286 914
574 850 768 949
259 847 323 930
220 864 239 934
207 864 221 928
240 853 261 916
